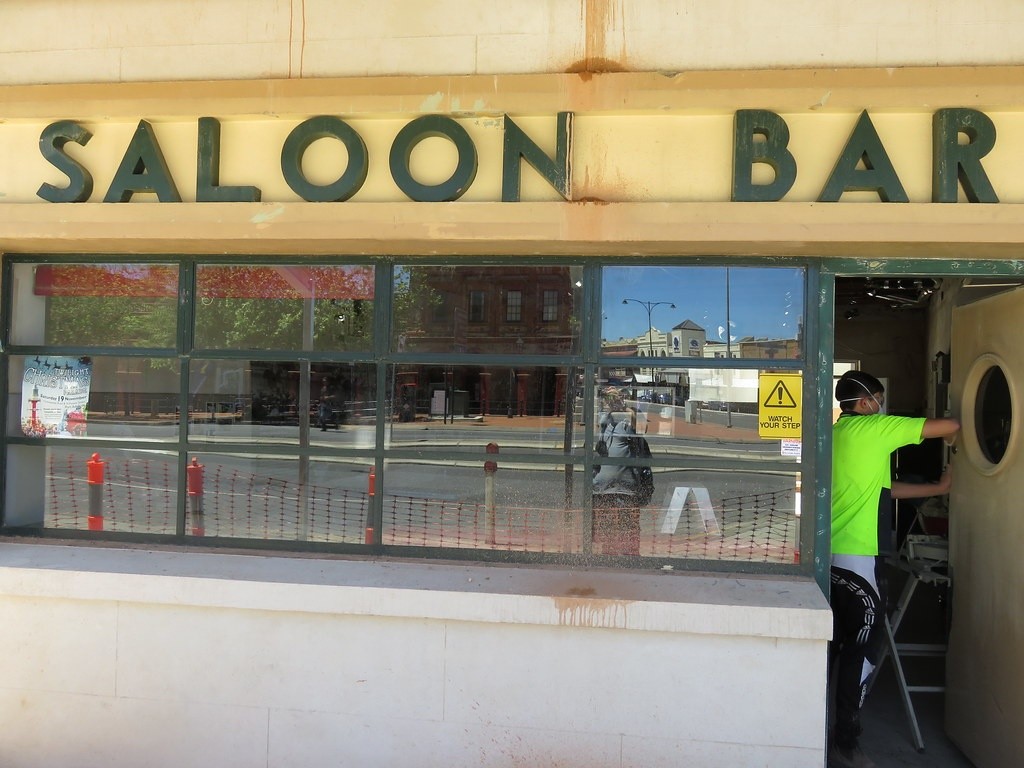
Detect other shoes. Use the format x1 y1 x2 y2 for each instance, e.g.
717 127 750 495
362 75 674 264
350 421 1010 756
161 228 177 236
336 424 338 429
320 428 326 431
832 740 876 766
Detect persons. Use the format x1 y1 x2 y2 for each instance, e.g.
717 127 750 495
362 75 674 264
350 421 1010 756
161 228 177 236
318 375 341 431
828 371 961 768
592 376 642 557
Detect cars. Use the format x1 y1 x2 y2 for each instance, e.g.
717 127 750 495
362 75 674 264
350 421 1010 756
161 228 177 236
640 394 757 414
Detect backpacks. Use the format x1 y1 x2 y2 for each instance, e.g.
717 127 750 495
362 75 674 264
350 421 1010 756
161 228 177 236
627 424 655 506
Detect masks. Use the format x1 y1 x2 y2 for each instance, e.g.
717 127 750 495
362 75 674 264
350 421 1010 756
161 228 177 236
840 378 884 414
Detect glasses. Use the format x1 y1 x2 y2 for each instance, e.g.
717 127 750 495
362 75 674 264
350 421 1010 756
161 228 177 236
321 381 328 384
868 394 884 406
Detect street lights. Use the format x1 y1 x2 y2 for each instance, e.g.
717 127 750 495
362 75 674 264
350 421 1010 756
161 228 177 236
622 298 676 402
